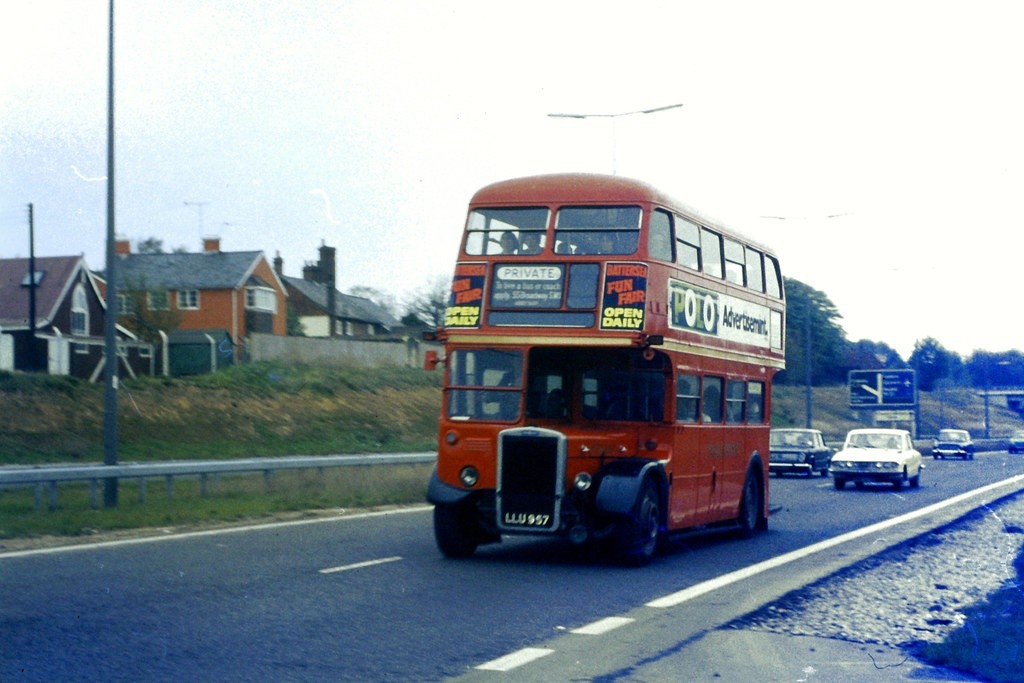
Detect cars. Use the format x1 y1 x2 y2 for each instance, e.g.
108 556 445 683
827 428 926 492
1007 430 1024 454
768 428 831 479
931 428 974 461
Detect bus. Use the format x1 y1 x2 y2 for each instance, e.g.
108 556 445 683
423 171 788 569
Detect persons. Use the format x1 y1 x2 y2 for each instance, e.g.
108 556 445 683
678 379 734 422
599 232 619 254
887 438 897 448
574 225 601 254
501 231 519 254
486 357 534 417
521 232 545 254
557 241 572 254
